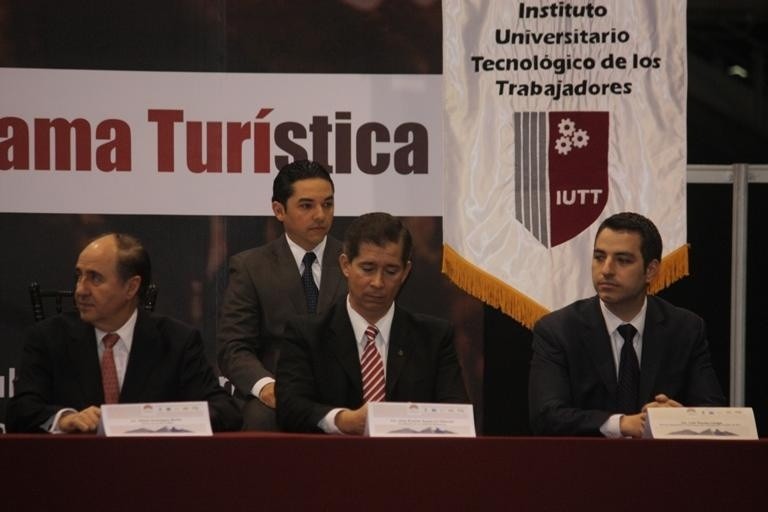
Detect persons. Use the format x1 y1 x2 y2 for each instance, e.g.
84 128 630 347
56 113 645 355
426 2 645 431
525 211 726 440
274 212 477 438
5 233 244 434
214 160 348 430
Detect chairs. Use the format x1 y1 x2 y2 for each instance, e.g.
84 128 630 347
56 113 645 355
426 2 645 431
29 280 158 321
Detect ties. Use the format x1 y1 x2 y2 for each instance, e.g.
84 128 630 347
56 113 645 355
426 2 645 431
616 323 640 415
301 252 319 316
360 324 386 403
102 333 120 403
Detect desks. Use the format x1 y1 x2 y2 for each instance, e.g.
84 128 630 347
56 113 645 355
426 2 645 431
0 430 768 512
